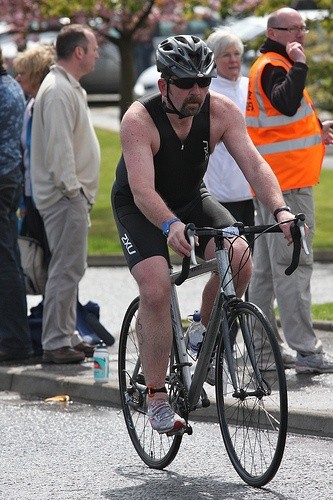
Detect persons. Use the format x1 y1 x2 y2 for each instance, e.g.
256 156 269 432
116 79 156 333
0 23 101 362
110 8 333 434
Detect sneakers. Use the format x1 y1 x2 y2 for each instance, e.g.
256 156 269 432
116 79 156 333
182 310 219 384
255 348 297 371
145 394 186 433
295 351 333 373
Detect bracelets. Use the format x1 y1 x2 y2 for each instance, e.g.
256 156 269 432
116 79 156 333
161 218 180 238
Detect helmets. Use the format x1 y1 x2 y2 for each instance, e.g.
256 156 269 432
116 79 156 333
155 34 219 78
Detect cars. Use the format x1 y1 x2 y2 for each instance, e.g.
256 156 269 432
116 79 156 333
132 8 328 104
1 13 213 94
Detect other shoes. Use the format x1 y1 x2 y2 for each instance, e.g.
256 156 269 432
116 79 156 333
75 340 96 358
42 347 84 365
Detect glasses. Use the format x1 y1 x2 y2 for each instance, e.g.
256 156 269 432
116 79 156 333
168 78 212 90
272 26 309 35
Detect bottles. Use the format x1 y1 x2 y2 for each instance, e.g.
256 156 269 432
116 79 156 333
188 314 206 374
93 340 109 383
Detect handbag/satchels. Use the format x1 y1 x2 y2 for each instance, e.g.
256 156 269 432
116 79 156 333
18 234 49 295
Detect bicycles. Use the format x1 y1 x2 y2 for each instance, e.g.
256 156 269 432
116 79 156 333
118 212 309 487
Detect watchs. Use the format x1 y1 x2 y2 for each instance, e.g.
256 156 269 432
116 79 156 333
273 205 290 222
